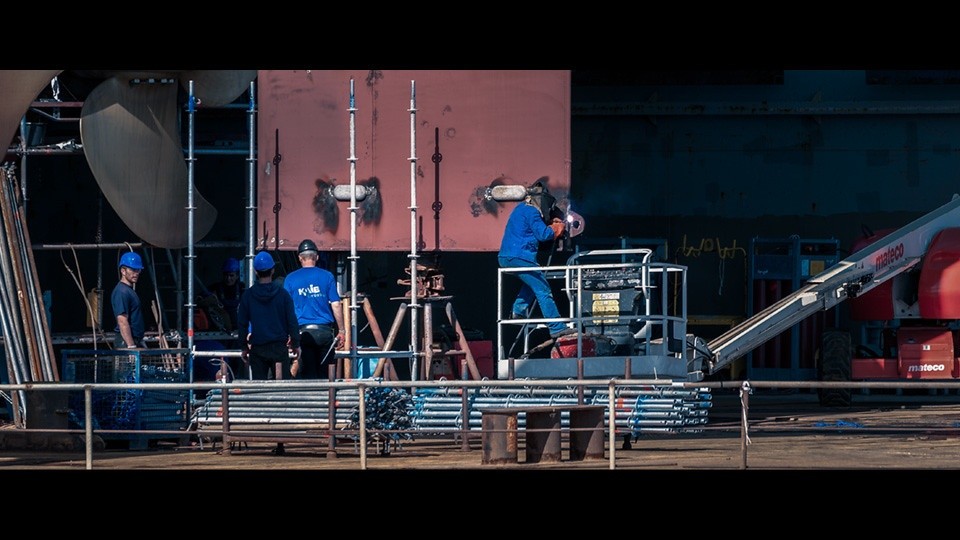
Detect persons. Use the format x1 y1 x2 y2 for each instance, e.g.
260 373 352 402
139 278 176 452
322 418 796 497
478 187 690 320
112 253 145 350
237 252 300 381
284 239 344 380
499 187 580 338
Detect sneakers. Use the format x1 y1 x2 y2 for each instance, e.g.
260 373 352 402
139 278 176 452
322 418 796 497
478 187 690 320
549 328 578 339
508 312 526 321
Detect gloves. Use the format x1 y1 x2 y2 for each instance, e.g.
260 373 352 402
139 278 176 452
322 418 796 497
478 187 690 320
334 331 346 351
548 217 565 240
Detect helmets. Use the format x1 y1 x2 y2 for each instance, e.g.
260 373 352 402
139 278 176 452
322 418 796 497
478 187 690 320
297 238 320 255
220 255 241 275
117 251 145 273
254 251 275 272
